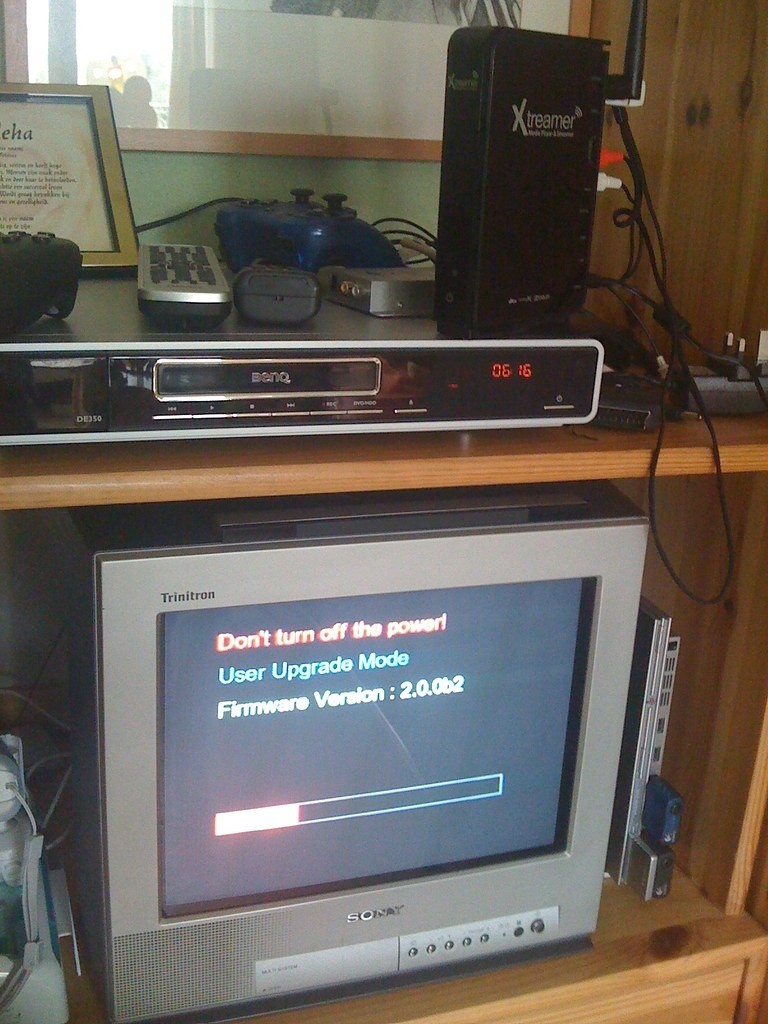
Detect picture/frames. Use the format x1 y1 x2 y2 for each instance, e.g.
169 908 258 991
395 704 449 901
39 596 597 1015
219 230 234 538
0 83 145 266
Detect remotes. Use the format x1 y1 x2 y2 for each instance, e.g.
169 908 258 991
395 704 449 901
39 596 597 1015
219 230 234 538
137 243 232 323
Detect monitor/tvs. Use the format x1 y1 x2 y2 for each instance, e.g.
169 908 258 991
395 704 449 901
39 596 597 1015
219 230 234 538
63 477 651 1024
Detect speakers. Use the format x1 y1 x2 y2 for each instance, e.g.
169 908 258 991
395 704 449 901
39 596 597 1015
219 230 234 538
435 26 608 330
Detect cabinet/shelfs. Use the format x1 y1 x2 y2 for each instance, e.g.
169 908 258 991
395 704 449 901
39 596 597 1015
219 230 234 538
0 418 768 1023
